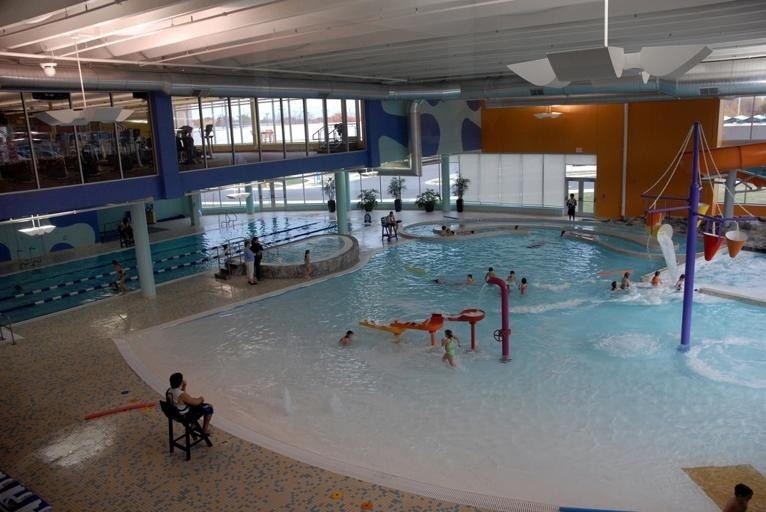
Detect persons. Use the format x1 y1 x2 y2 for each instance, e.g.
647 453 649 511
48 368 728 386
466 267 528 291
567 193 577 221
166 372 213 436
440 226 455 237
652 271 663 286
675 275 685 291
120 218 133 240
244 237 264 286
112 260 127 295
724 483 754 511
13 285 23 293
441 330 460 366
386 212 396 237
304 249 312 282
611 272 632 292
338 330 354 346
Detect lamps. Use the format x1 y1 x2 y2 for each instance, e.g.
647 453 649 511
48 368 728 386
16 214 59 238
38 60 58 77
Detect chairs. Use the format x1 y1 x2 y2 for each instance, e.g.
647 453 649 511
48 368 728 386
116 224 135 248
157 397 215 463
379 214 399 243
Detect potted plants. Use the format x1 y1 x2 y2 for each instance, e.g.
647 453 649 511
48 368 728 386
355 188 381 213
322 178 339 214
451 175 470 213
387 177 408 213
413 187 442 214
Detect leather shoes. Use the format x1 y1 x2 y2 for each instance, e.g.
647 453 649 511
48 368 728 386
248 281 257 285
